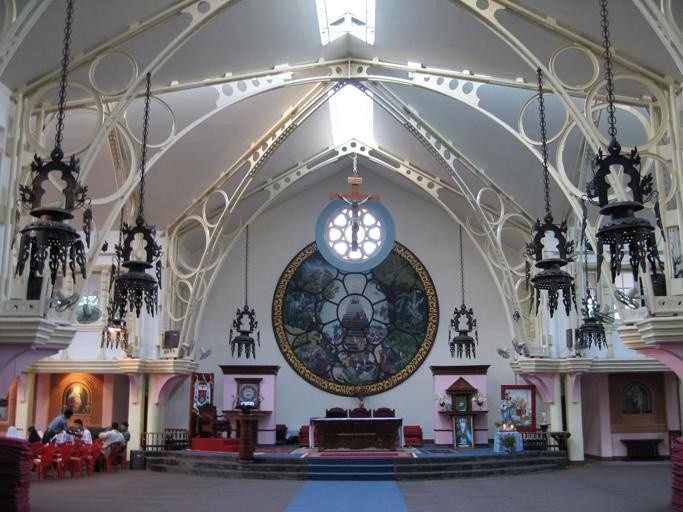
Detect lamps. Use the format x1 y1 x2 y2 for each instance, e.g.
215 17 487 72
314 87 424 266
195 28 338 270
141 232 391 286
228 225 260 360
447 224 478 358
577 0 667 285
523 66 579 316
100 73 160 352
10 0 93 288
572 236 608 350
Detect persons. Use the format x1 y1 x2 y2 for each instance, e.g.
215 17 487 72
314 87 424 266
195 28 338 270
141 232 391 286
26 425 41 442
60 419 93 470
624 385 635 411
113 423 130 454
336 192 373 246
632 387 645 412
28 408 80 477
93 422 126 472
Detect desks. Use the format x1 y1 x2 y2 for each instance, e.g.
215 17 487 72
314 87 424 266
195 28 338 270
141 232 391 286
620 440 665 461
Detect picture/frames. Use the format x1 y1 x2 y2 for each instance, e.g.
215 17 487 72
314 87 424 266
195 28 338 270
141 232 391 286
61 381 92 416
234 378 262 410
501 385 536 432
452 414 474 448
622 381 652 414
190 372 214 416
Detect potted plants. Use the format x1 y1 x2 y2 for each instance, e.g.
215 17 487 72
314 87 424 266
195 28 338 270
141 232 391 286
501 437 516 455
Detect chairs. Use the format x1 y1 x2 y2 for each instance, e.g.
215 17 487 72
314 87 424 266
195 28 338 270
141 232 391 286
523 430 548 450
198 404 232 439
28 436 130 480
274 407 424 448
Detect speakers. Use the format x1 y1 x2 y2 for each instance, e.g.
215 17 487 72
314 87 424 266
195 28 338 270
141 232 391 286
130 450 146 470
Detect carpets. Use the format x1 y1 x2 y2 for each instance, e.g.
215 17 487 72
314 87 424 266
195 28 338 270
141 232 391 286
301 450 416 458
185 448 294 453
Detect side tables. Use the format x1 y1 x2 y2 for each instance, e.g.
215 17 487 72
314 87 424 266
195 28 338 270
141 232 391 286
493 432 524 452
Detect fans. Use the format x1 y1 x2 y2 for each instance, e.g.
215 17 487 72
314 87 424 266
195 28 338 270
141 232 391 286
75 303 102 324
183 339 195 356
512 338 527 354
614 287 648 309
496 347 512 359
199 346 211 360
53 288 79 312
590 304 619 323
